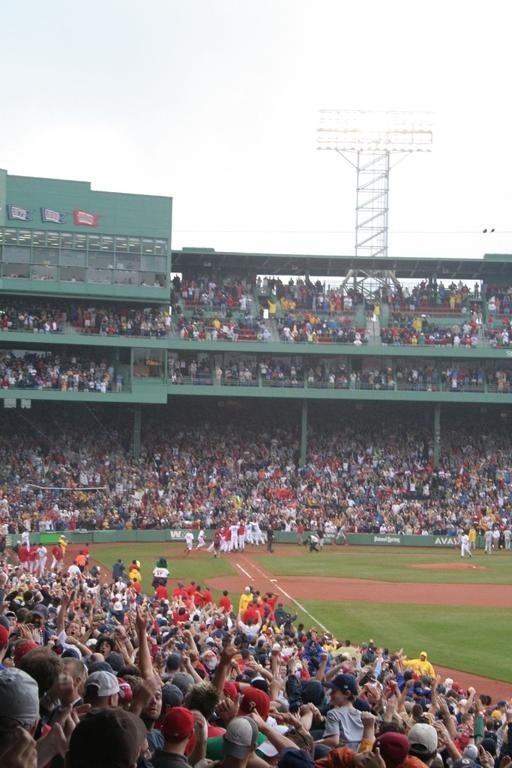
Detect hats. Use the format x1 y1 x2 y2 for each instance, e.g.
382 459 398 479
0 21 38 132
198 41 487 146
0 562 509 768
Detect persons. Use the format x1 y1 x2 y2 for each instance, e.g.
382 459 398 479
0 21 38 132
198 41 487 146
0 605 511 767
2 268 510 556
0 556 278 606
